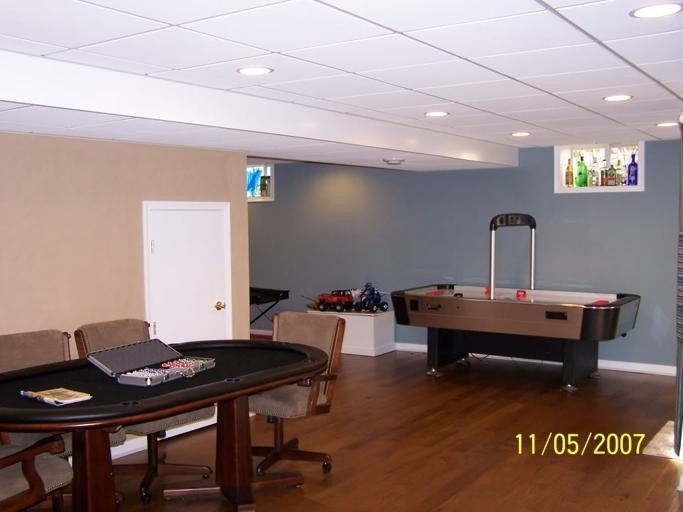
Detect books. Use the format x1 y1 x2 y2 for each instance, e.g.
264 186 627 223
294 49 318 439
19 387 95 407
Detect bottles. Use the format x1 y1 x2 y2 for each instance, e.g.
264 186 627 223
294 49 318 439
259 175 273 197
565 153 639 186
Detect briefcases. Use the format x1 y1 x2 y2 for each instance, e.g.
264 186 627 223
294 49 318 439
85 339 215 387
